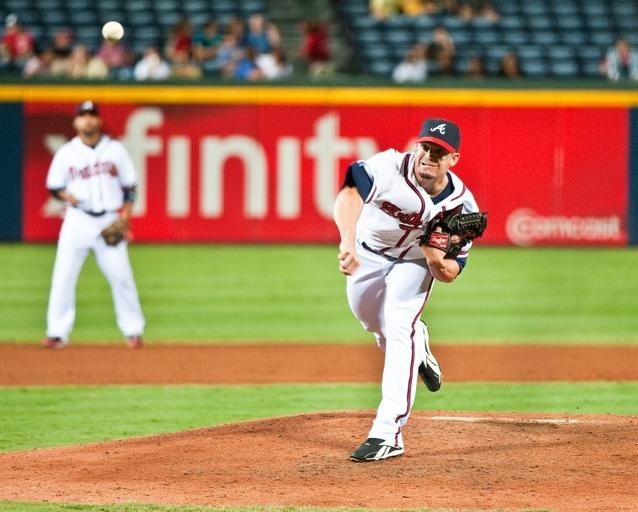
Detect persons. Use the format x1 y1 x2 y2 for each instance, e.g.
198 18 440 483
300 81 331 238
42 99 146 349
391 25 525 83
367 1 499 22
1 12 335 79
598 36 638 78
323 115 481 460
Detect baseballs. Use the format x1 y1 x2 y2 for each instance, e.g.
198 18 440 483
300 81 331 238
102 22 124 41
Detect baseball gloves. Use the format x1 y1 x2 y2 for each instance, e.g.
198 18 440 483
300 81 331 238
419 212 487 258
101 219 128 244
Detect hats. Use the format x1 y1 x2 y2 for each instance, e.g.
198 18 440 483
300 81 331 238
415 118 460 153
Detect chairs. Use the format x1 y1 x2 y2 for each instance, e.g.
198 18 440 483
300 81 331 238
0 0 638 79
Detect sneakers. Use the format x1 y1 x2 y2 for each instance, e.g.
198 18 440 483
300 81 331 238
419 320 441 391
350 438 404 462
